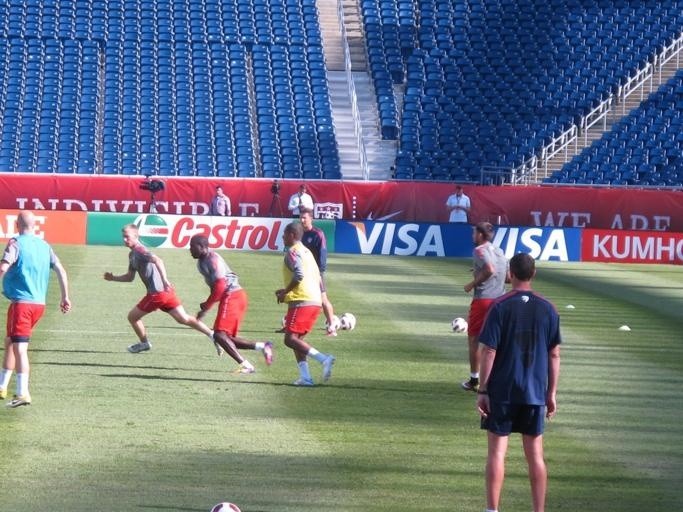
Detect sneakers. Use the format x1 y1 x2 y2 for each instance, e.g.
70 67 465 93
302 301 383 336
294 377 312 386
212 335 224 357
263 341 274 365
322 355 336 383
6 397 31 407
0 388 6 398
275 327 285 332
463 381 478 390
325 327 336 336
230 367 253 373
128 344 150 353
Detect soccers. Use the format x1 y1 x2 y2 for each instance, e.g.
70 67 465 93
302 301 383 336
211 501 241 512
338 312 356 331
324 316 341 331
452 317 468 334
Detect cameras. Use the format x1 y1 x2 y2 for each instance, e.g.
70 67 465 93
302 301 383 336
140 174 164 191
271 179 280 193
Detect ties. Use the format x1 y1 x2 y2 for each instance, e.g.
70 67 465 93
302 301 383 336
457 196 460 211
299 195 302 213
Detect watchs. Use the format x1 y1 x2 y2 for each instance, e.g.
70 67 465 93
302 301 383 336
477 390 487 395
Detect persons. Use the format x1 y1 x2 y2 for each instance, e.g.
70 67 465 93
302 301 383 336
288 184 313 219
476 253 563 512
295 209 336 336
209 186 231 216
446 185 471 223
189 235 273 374
461 223 511 390
0 210 71 408
103 224 224 357
275 222 334 385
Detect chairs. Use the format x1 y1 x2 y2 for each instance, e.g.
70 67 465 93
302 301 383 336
0 0 342 177
368 0 683 189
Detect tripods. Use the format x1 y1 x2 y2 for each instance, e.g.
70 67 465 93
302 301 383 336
146 192 160 213
269 192 285 217
